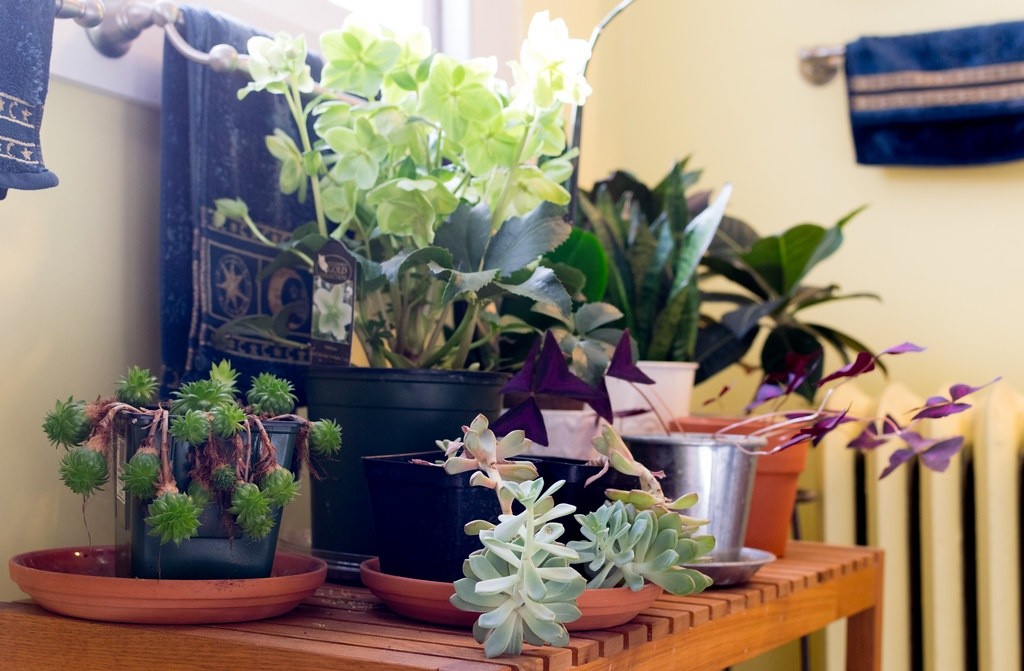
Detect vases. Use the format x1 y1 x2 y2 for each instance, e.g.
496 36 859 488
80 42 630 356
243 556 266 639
307 365 505 587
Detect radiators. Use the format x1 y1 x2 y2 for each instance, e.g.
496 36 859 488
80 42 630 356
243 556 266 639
806 376 1022 669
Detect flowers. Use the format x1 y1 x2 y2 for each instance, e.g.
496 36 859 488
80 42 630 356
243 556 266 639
210 7 590 370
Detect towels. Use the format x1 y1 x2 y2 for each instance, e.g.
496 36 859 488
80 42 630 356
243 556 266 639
0 1 58 204
843 21 1023 168
156 5 381 400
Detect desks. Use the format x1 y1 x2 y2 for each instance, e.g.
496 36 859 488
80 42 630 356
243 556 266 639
2 542 885 671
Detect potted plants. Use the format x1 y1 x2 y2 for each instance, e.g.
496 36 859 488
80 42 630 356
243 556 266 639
571 154 735 433
483 327 1002 585
670 205 889 559
40 356 343 580
381 411 717 659
